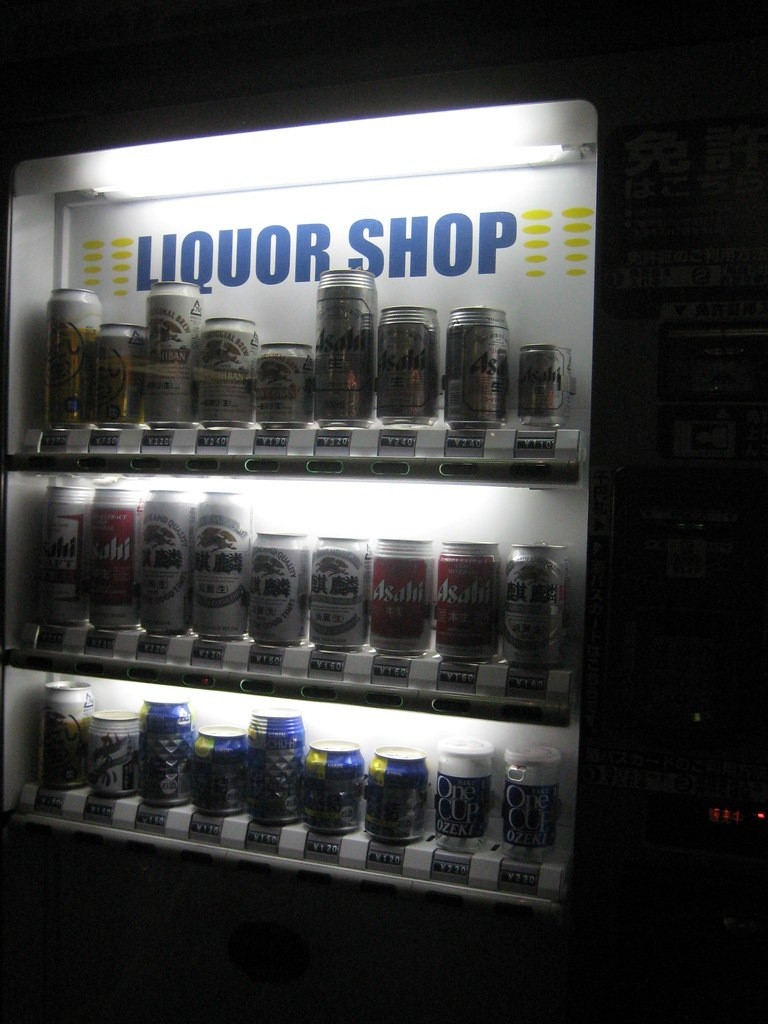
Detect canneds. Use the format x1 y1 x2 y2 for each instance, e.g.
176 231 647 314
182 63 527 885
30 269 575 861
